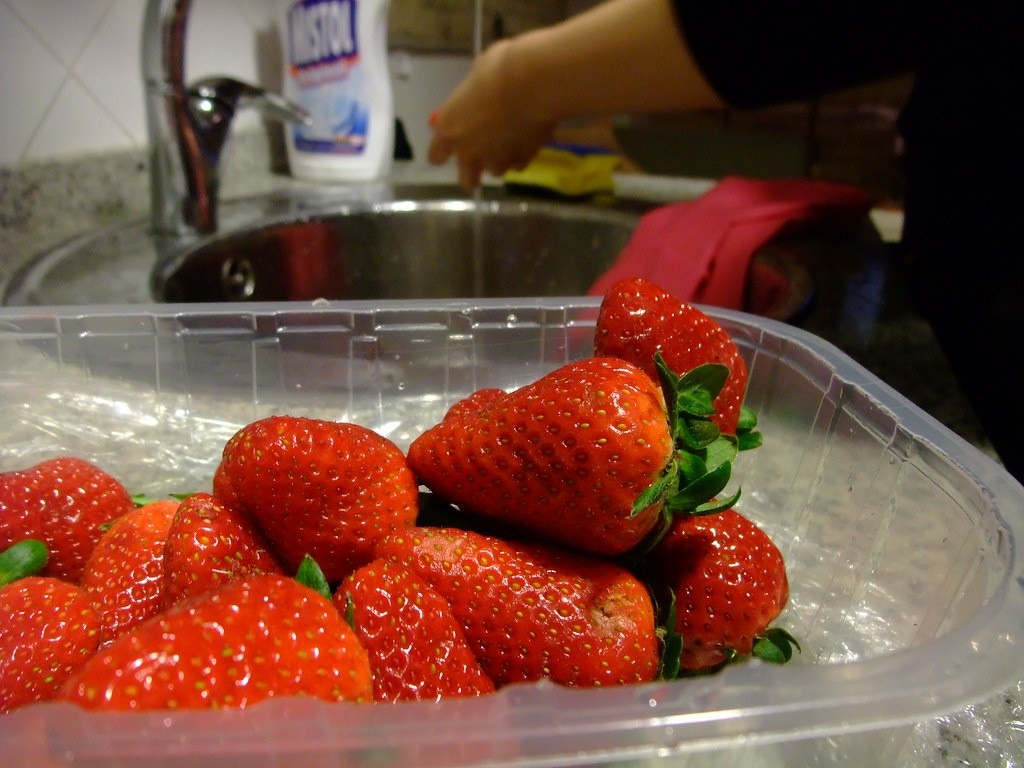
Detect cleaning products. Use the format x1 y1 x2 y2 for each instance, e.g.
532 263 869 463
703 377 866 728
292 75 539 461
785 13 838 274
276 0 395 209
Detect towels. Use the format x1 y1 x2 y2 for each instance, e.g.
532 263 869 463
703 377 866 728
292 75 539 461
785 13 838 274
542 174 883 367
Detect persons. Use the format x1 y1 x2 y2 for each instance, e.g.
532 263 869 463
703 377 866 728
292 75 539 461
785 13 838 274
425 1 1024 486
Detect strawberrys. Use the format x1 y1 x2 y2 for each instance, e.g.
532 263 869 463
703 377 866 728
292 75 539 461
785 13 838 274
0 275 801 716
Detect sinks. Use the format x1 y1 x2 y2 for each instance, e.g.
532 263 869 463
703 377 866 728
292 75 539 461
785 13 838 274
1 177 849 411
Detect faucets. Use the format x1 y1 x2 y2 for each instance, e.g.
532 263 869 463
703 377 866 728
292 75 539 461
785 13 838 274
133 0 320 241
